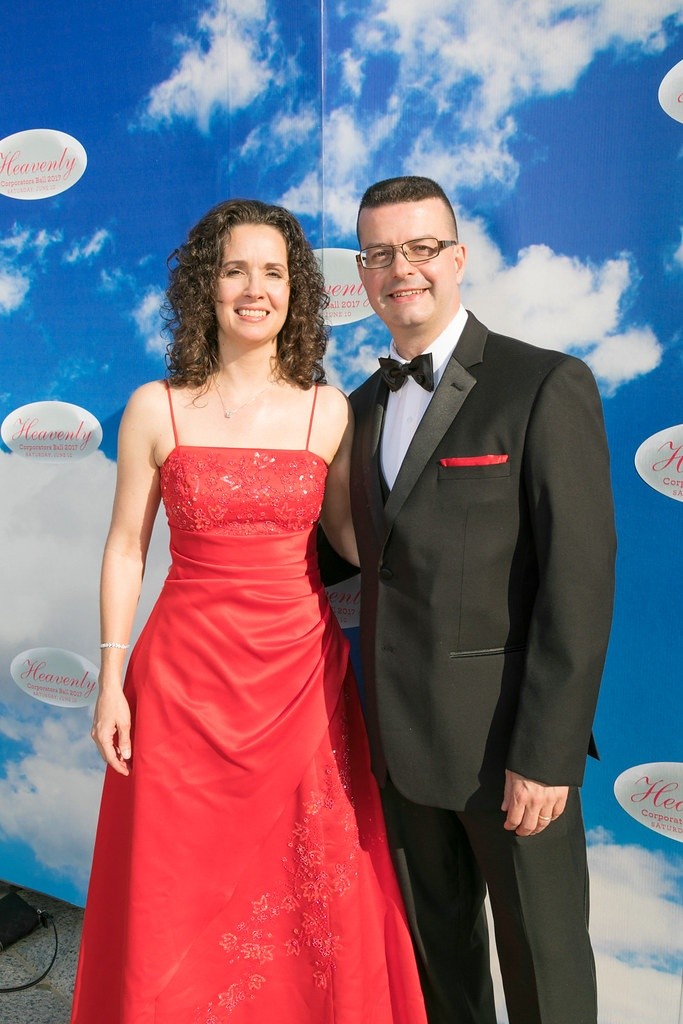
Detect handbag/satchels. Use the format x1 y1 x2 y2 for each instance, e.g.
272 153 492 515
0 892 57 993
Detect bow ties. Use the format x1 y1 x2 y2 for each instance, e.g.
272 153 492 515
378 352 435 392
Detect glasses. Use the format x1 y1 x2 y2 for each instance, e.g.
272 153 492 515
356 237 457 270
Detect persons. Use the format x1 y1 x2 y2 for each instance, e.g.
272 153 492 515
348 175 618 1024
70 200 430 1023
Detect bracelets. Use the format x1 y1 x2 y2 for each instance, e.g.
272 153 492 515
99 643 129 649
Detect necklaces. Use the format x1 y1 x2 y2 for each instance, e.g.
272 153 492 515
212 373 278 418
